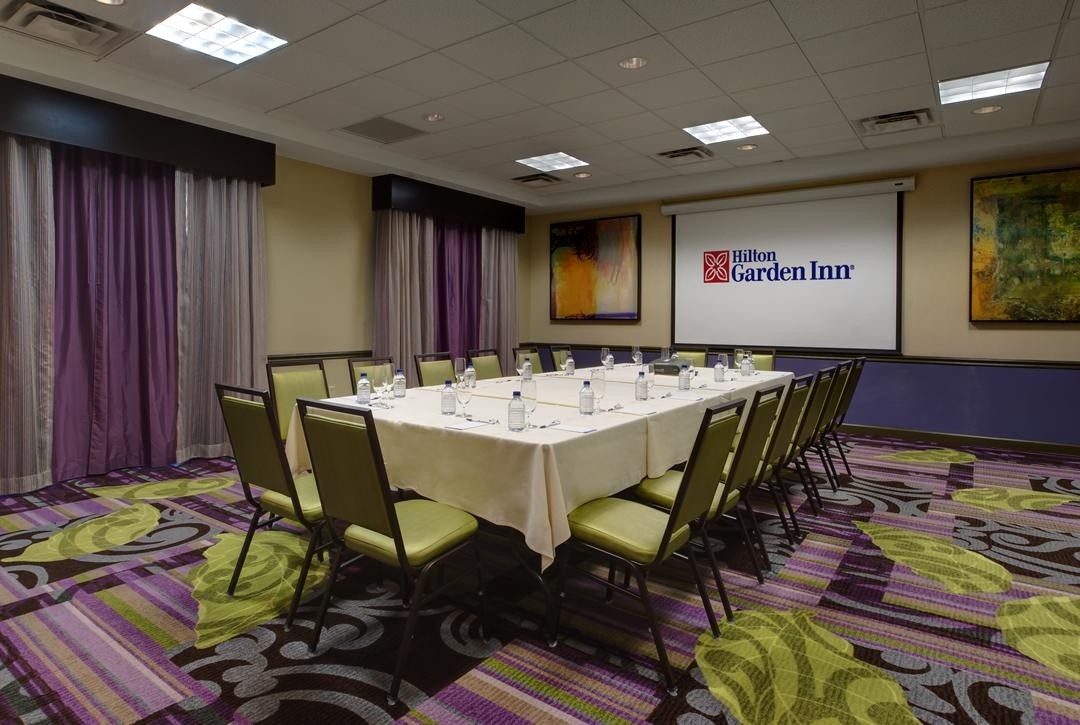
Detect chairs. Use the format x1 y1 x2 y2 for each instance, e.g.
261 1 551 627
265 358 333 444
468 347 506 381
411 350 460 388
554 354 867 695
733 347 777 371
669 344 710 368
551 344 576 373
212 380 391 634
295 398 491 710
510 342 547 373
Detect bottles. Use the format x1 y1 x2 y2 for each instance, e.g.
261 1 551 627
357 343 752 431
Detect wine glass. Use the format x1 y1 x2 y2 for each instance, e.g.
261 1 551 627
371 344 752 428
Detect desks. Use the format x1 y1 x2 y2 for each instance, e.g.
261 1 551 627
277 357 798 643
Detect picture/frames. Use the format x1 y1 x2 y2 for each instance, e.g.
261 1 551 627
549 211 640 324
969 166 1080 325
